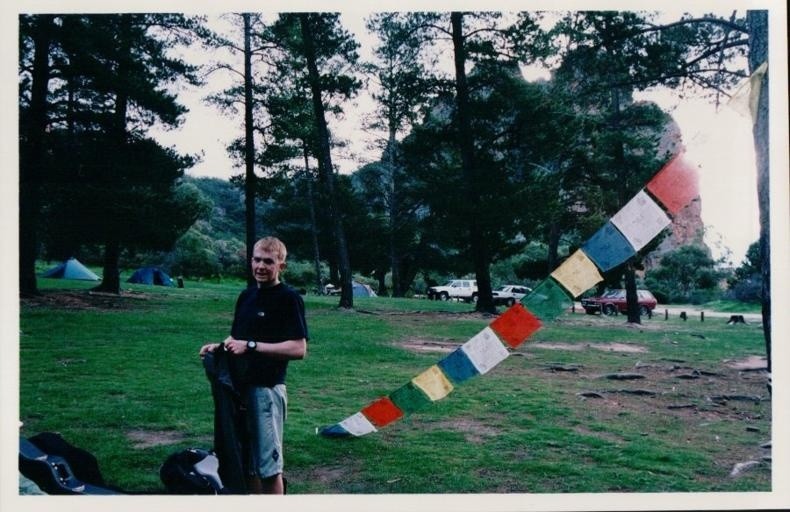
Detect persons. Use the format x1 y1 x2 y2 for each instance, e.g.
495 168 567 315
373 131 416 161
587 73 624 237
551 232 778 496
199 236 311 494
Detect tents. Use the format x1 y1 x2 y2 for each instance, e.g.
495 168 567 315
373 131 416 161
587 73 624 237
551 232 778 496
127 266 176 287
352 280 378 298
35 256 104 281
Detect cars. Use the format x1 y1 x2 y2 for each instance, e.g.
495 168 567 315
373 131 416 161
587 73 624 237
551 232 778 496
492 284 549 307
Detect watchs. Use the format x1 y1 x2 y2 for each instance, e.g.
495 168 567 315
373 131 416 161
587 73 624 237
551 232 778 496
246 340 256 351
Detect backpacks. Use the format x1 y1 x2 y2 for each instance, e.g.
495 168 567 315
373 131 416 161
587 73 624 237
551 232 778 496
158 449 221 494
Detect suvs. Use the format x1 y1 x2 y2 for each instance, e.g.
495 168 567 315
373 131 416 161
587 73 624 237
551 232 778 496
580 288 657 316
429 279 479 301
